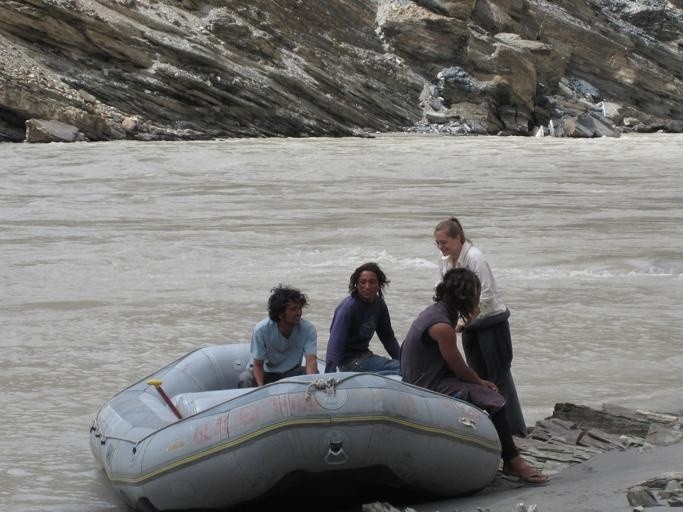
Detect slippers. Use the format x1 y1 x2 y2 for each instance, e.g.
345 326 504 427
501 469 551 486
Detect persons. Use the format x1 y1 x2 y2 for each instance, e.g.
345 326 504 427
236 282 321 389
323 261 401 376
398 260 552 488
433 215 530 436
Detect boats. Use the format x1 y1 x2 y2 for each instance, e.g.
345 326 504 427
82 338 507 512
